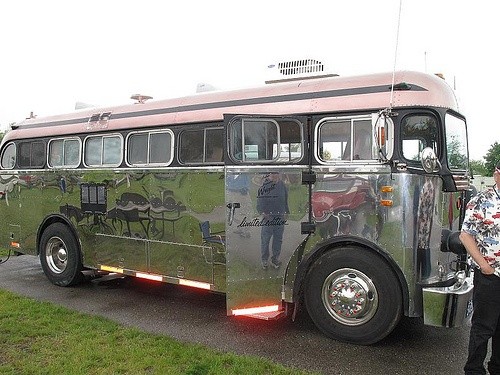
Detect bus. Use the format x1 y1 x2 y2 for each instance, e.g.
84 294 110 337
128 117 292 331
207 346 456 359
0 54 477 346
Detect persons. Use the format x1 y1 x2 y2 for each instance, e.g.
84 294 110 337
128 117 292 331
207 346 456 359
255 172 291 270
458 159 500 375
414 175 434 282
